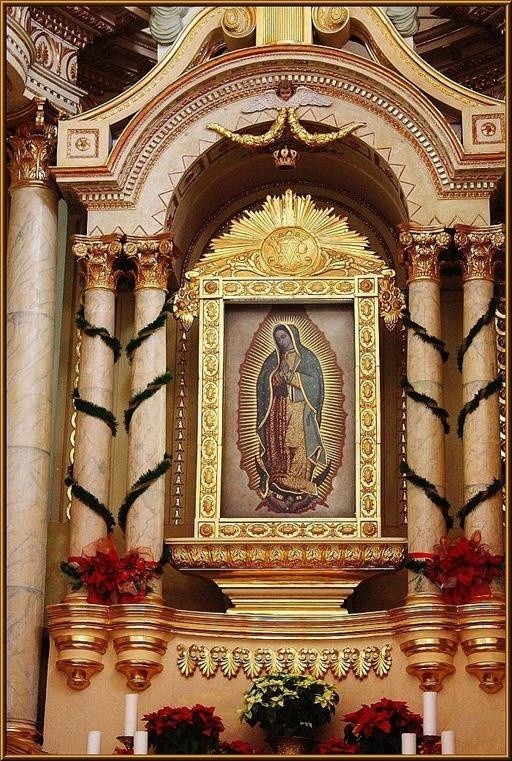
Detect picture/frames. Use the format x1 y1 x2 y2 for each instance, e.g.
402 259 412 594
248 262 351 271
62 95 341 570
181 737 200 752
197 272 380 539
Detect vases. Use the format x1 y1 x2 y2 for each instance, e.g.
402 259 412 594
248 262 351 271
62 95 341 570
373 735 391 753
274 731 311 756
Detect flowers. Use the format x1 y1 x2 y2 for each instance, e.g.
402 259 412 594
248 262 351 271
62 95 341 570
340 697 424 739
140 704 225 744
238 670 339 733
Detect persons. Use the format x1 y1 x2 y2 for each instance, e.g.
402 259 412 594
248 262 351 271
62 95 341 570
255 323 327 501
276 79 294 100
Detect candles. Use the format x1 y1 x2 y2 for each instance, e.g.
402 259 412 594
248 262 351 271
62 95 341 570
132 730 149 757
88 730 102 756
125 692 139 736
440 730 454 753
422 692 436 737
399 730 416 754
